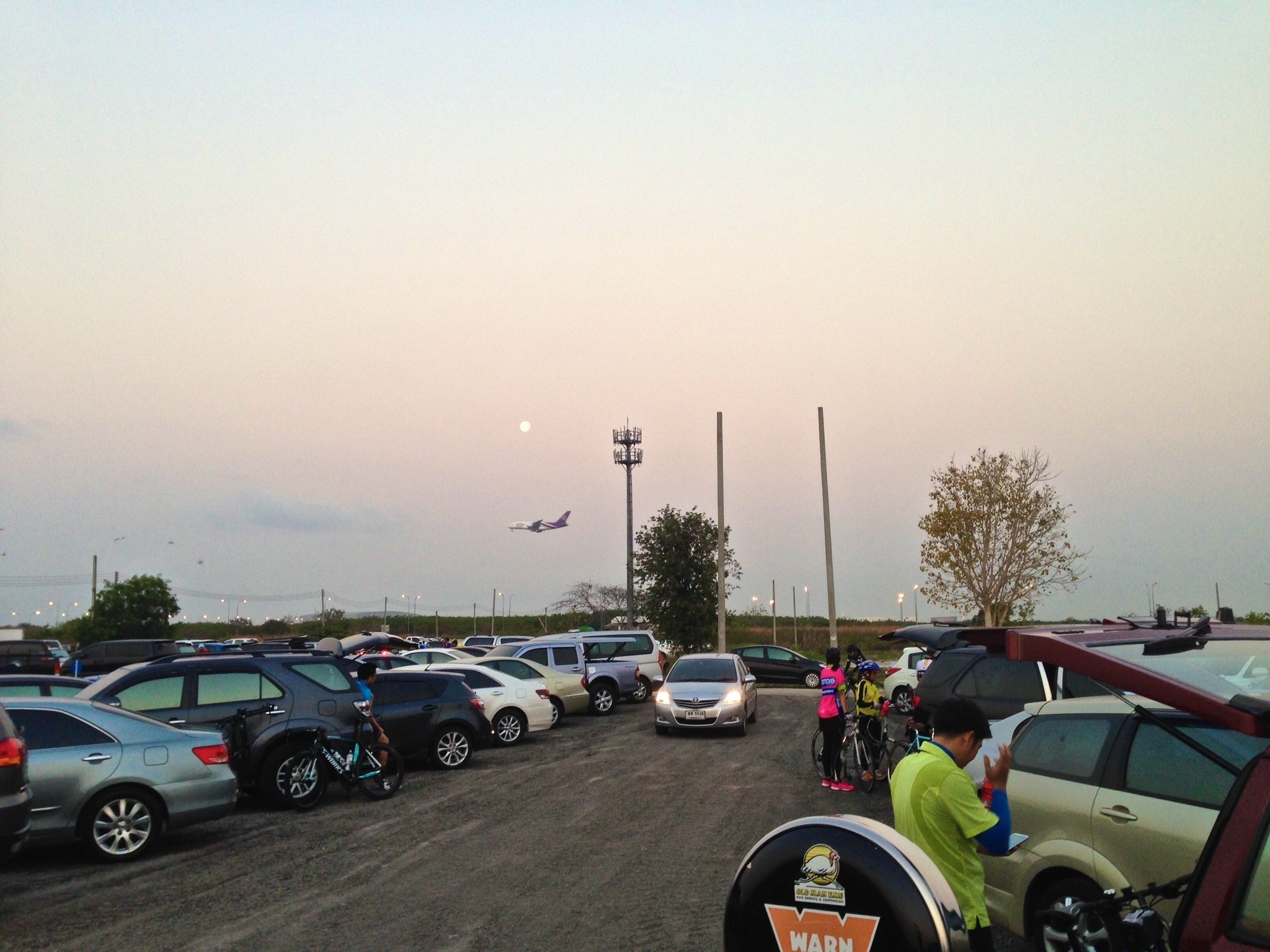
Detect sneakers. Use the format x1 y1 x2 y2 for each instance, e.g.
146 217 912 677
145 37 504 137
831 779 854 790
821 779 835 786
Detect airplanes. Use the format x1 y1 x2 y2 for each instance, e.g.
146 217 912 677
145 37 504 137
508 510 572 533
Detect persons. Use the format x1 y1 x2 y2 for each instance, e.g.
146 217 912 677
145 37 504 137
818 647 855 791
843 643 866 684
354 662 390 790
891 698 1021 952
916 645 936 680
418 637 464 649
856 660 888 781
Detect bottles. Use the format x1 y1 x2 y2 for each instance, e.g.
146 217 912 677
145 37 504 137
880 699 889 716
981 775 994 809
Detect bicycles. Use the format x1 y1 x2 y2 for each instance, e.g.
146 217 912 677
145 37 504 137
843 670 885 714
874 716 934 789
1032 872 1193 952
284 714 405 811
811 711 876 794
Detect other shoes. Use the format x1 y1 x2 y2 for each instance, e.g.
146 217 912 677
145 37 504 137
875 770 887 780
862 771 873 781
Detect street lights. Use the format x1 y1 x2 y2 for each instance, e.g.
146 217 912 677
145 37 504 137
237 600 247 620
66 602 77 623
752 596 758 616
1152 583 1157 616
499 592 504 617
324 597 331 621
804 586 810 626
1146 583 1152 617
49 601 59 628
402 595 410 633
221 599 230 624
913 584 918 623
898 593 904 622
1025 584 1033 626
509 594 516 617
414 596 420 636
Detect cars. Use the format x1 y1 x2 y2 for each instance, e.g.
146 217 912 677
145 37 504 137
652 652 756 737
879 616 1270 952
1 630 662 865
728 644 827 689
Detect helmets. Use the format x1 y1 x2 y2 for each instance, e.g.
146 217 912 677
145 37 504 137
315 637 343 657
858 660 881 672
